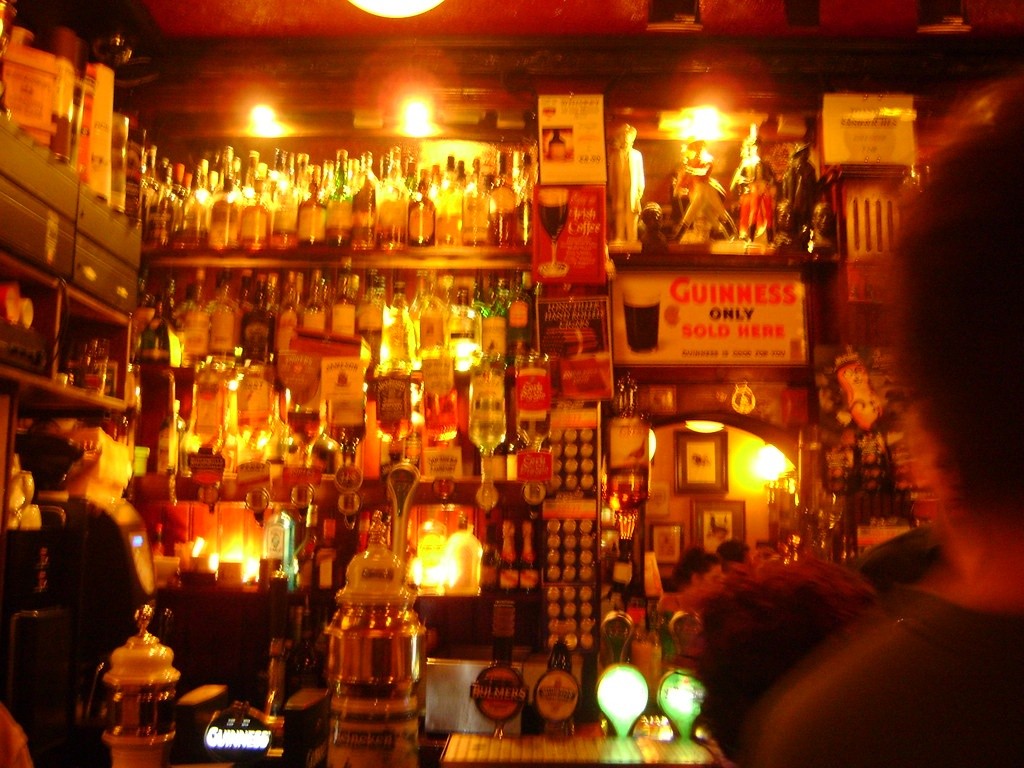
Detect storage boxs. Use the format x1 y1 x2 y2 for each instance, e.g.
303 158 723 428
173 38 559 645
0 174 75 277
77 180 142 268
72 232 134 316
0 110 78 220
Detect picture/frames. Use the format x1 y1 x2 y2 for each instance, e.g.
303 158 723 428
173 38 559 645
649 520 684 565
671 427 729 494
691 497 746 553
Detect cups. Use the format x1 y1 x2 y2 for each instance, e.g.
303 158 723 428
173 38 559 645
620 276 661 352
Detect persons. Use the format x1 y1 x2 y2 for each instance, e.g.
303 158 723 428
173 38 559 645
659 535 790 627
1 419 152 768
608 117 833 253
734 75 1024 768
692 562 877 744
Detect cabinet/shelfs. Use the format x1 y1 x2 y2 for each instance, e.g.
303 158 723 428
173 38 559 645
135 122 542 734
0 252 130 417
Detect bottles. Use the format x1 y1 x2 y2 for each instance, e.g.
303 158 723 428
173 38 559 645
605 372 647 554
143 140 550 594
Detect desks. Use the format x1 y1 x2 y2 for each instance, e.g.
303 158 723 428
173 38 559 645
439 714 731 768
